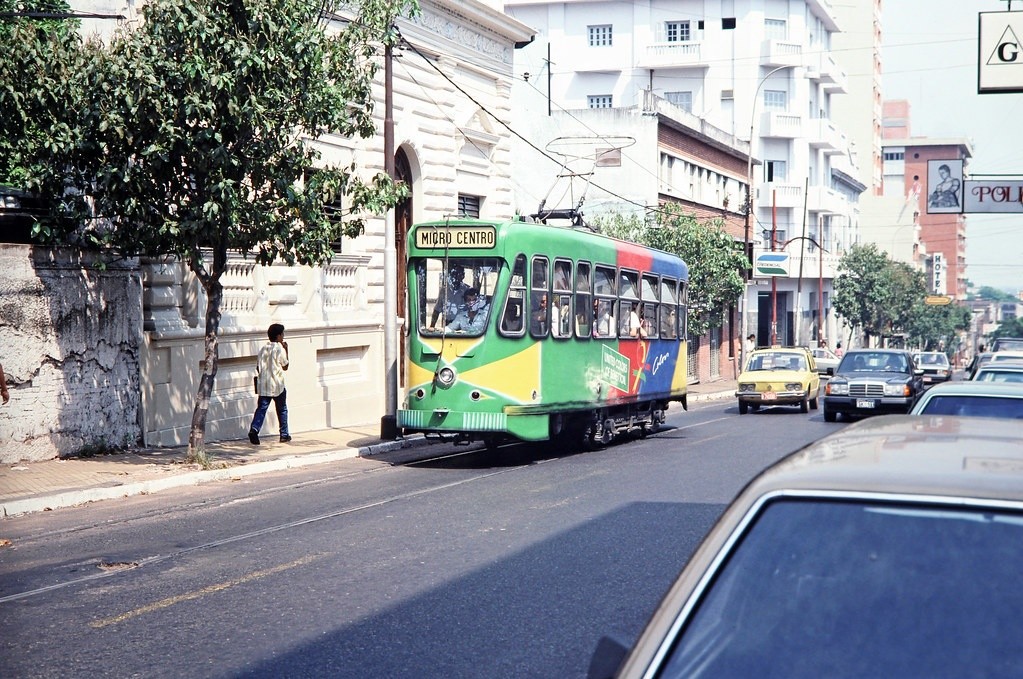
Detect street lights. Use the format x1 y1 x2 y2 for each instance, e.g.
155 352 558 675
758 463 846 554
740 64 819 372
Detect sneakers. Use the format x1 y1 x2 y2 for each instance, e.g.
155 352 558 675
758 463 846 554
280 435 291 442
248 429 260 444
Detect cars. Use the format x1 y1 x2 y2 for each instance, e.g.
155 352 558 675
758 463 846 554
905 380 1023 419
734 345 821 413
809 348 842 375
911 336 1023 385
823 349 927 421
587 415 1023 679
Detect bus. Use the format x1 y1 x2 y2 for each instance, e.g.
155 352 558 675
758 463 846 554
396 208 691 459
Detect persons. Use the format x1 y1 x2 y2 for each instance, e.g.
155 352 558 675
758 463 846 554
505 293 684 339
248 323 291 444
746 334 756 353
852 357 866 369
428 265 470 332
835 344 842 357
884 355 905 373
932 356 944 365
785 358 804 372
950 356 968 368
931 165 962 206
439 288 488 334
820 342 828 357
